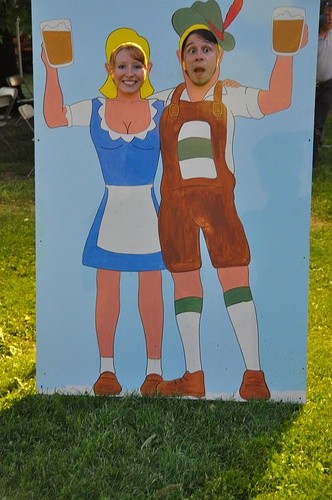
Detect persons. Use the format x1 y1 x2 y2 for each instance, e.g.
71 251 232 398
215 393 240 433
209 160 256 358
0 24 24 114
109 44 149 94
181 29 218 86
313 0 332 161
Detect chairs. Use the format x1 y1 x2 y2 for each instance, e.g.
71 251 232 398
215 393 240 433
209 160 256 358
0 74 34 177
